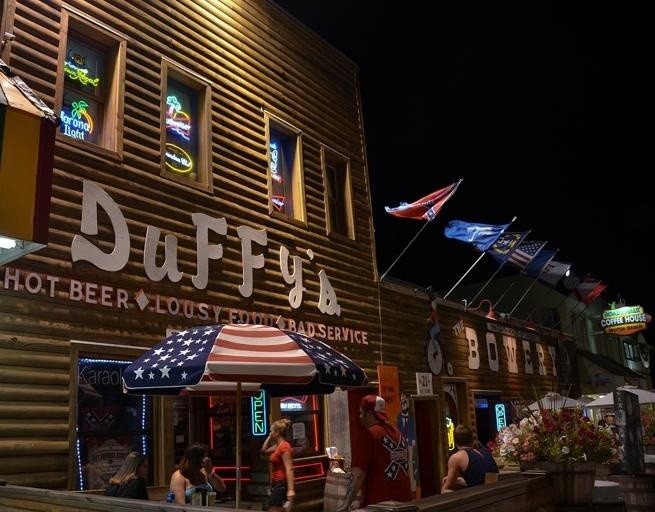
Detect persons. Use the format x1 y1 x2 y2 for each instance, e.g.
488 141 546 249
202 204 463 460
259 418 296 512
440 423 500 494
335 394 413 512
396 393 417 498
169 441 227 505
103 451 150 500
471 439 500 473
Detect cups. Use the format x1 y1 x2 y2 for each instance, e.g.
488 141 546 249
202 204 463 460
166 492 175 504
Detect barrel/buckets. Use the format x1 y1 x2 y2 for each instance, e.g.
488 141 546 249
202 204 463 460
543 462 596 512
324 467 362 511
618 473 655 512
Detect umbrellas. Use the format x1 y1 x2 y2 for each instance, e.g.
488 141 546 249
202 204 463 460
122 323 369 505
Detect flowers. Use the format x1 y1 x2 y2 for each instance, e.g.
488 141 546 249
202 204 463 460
506 411 622 468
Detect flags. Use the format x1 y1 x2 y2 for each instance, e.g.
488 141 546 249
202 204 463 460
486 231 608 307
443 220 509 252
383 182 458 222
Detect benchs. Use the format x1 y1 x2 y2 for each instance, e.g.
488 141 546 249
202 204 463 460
10 455 562 510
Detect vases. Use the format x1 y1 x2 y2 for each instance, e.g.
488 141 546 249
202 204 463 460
555 461 597 503
